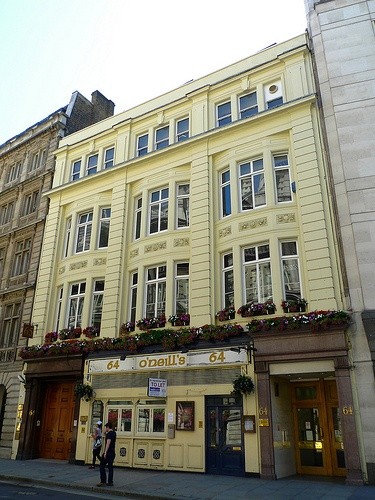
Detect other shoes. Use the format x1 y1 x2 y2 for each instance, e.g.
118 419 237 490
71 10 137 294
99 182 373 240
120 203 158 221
106 483 113 486
88 466 96 469
97 483 106 487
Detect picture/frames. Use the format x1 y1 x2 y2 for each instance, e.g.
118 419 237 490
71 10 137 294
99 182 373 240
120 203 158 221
175 401 195 432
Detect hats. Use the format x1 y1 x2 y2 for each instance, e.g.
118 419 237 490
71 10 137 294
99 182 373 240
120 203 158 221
96 421 102 425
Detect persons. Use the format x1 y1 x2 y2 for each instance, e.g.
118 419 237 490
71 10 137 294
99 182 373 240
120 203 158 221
88 421 102 468
96 423 116 487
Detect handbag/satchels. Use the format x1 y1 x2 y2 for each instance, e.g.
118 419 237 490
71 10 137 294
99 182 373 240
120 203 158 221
94 428 102 448
